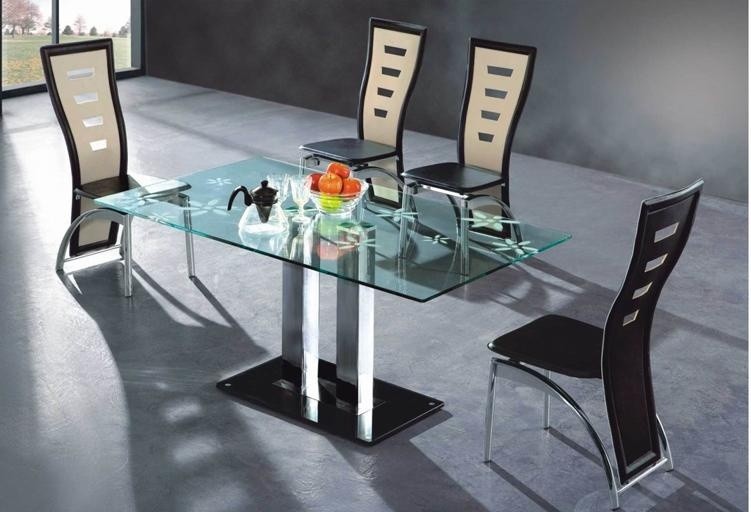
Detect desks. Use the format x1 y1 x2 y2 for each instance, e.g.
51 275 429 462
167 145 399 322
90 152 574 450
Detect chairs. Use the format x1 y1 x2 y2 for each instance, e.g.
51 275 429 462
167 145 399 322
38 39 195 298
296 16 429 228
481 177 707 512
397 36 540 279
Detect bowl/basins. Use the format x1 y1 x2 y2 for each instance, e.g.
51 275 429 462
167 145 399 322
306 179 369 215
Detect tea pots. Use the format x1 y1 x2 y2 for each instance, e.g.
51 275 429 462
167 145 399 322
228 180 290 234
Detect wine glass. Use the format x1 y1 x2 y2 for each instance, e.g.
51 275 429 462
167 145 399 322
266 176 289 222
290 175 312 224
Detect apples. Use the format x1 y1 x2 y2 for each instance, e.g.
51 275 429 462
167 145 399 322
318 173 342 193
340 179 361 198
326 163 350 179
307 173 322 191
